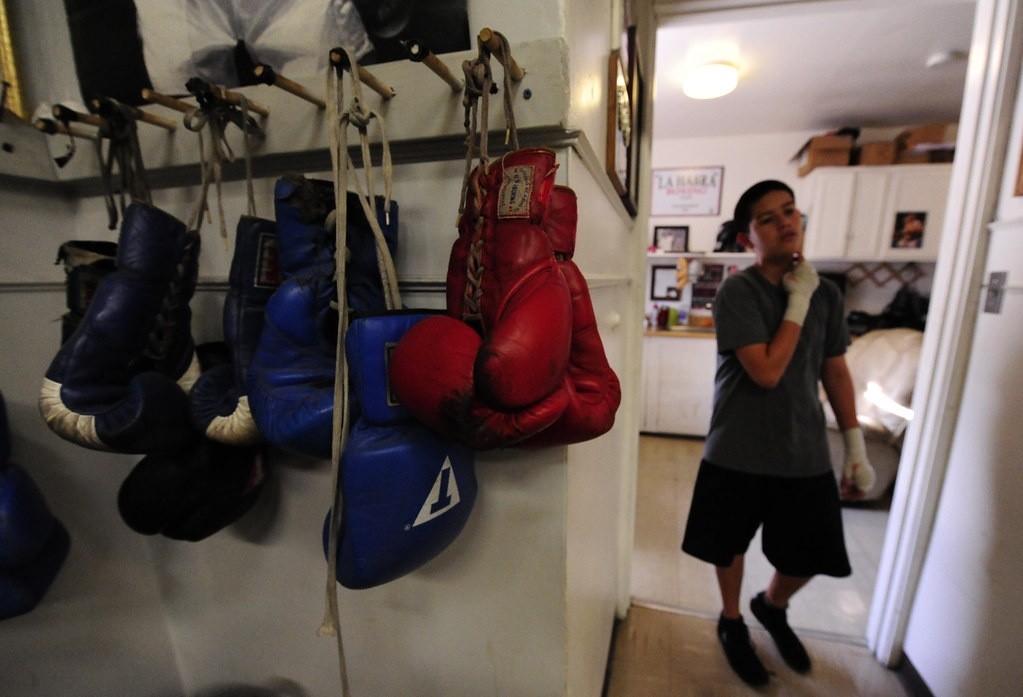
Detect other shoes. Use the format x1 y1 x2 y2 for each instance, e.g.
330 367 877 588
716 613 770 687
750 590 809 674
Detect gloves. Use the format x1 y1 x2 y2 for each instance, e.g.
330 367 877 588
117 218 272 540
322 310 476 590
390 148 621 450
247 177 397 458
40 208 197 452
0 392 71 619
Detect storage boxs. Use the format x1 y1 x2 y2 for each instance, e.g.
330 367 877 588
789 134 895 177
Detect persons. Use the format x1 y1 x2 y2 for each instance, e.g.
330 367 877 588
681 181 875 686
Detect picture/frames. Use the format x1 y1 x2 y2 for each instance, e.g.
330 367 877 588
653 225 689 252
651 263 681 301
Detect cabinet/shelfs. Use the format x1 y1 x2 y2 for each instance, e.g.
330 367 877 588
640 336 717 436
798 166 891 259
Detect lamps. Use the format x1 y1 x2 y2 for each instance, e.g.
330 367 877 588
925 51 969 73
682 64 741 100
